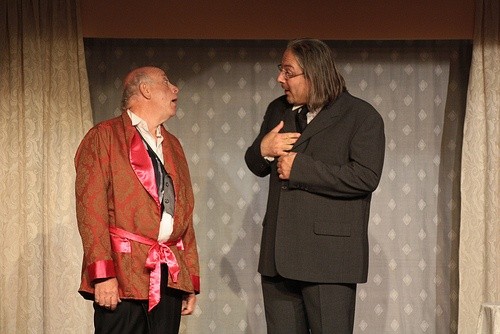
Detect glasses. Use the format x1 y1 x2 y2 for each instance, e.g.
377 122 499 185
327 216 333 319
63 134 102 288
277 64 306 80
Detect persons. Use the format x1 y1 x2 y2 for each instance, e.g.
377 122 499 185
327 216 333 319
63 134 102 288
74 67 200 334
243 39 385 334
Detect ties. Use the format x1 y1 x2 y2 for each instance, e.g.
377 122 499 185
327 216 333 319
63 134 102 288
295 104 309 136
